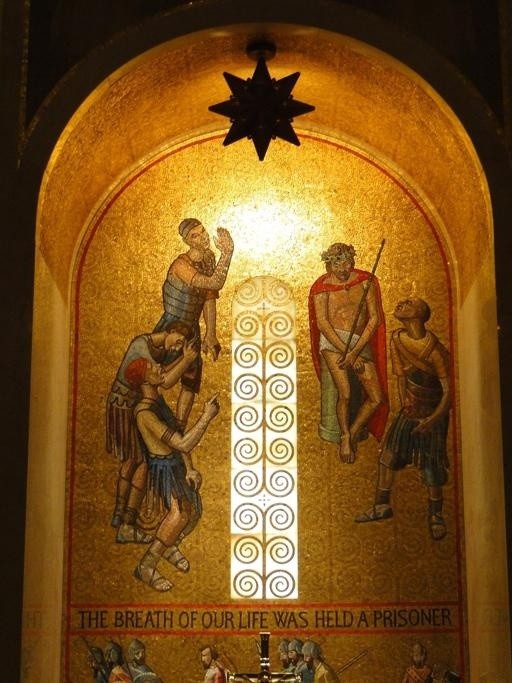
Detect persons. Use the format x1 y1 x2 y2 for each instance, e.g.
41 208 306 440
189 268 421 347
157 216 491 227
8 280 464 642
104 320 203 544
122 358 223 593
150 217 236 435
306 242 386 466
353 295 452 541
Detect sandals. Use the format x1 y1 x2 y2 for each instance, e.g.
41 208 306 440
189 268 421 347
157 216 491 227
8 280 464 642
427 513 447 541
110 512 154 544
163 548 190 574
134 562 175 595
353 506 393 523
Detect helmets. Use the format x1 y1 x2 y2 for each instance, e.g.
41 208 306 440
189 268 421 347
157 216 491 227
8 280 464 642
277 639 291 655
287 639 304 657
301 640 324 661
84 638 147 670
